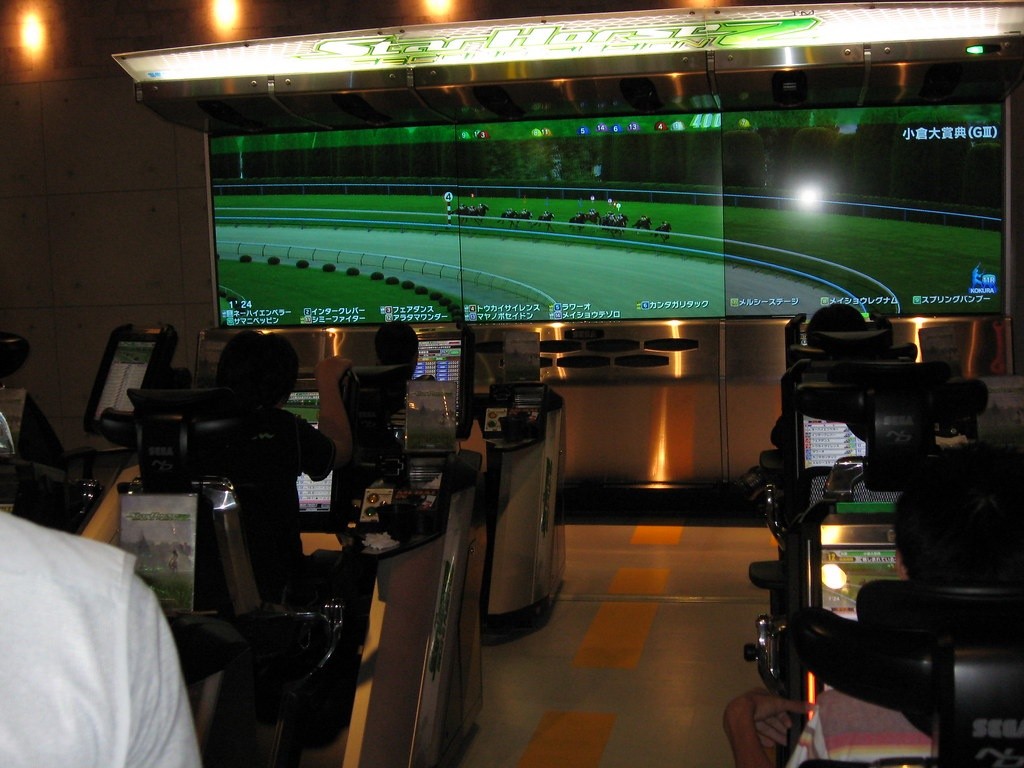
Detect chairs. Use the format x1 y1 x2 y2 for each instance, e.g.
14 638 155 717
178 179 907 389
0 310 1024 767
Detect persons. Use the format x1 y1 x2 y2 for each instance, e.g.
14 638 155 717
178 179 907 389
0 321 418 768
722 304 1024 768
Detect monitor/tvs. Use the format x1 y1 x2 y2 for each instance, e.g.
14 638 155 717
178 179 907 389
81 324 470 620
793 378 911 724
